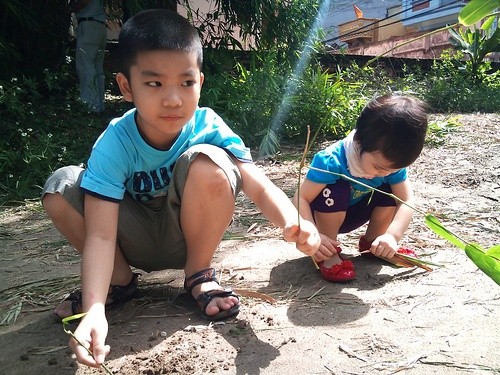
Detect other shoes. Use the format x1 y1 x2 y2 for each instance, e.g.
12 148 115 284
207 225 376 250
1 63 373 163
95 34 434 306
315 246 357 282
359 235 418 268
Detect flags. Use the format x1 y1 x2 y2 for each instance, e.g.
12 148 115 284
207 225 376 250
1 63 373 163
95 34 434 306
354 5 363 19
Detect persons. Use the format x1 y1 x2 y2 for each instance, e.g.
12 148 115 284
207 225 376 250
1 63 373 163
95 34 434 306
75 0 107 118
293 94 428 281
41 10 321 369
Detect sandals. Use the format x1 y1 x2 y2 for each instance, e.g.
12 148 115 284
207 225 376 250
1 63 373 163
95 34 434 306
184 268 240 320
54 273 143 322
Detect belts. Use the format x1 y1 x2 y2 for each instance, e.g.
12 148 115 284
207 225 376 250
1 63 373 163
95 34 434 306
78 17 112 29
